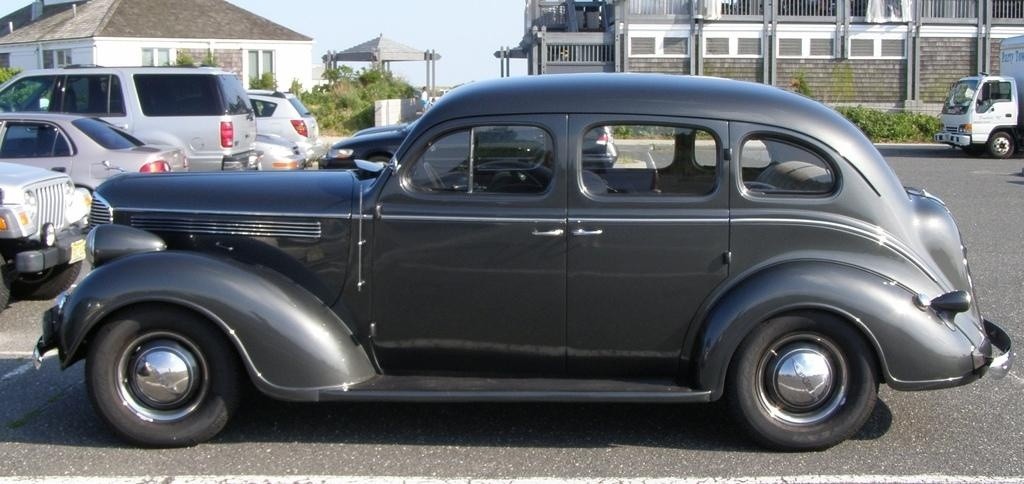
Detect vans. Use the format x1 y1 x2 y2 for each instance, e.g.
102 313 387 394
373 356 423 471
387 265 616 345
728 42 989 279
5 66 265 174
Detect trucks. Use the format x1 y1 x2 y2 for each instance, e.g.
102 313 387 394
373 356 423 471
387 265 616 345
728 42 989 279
934 36 1024 157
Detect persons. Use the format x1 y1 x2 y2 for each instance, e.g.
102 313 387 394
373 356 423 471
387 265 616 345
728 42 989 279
415 86 428 113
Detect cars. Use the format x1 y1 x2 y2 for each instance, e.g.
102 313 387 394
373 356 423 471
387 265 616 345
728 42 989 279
256 128 308 172
245 87 320 170
0 110 187 233
27 71 1012 452
1 155 97 312
